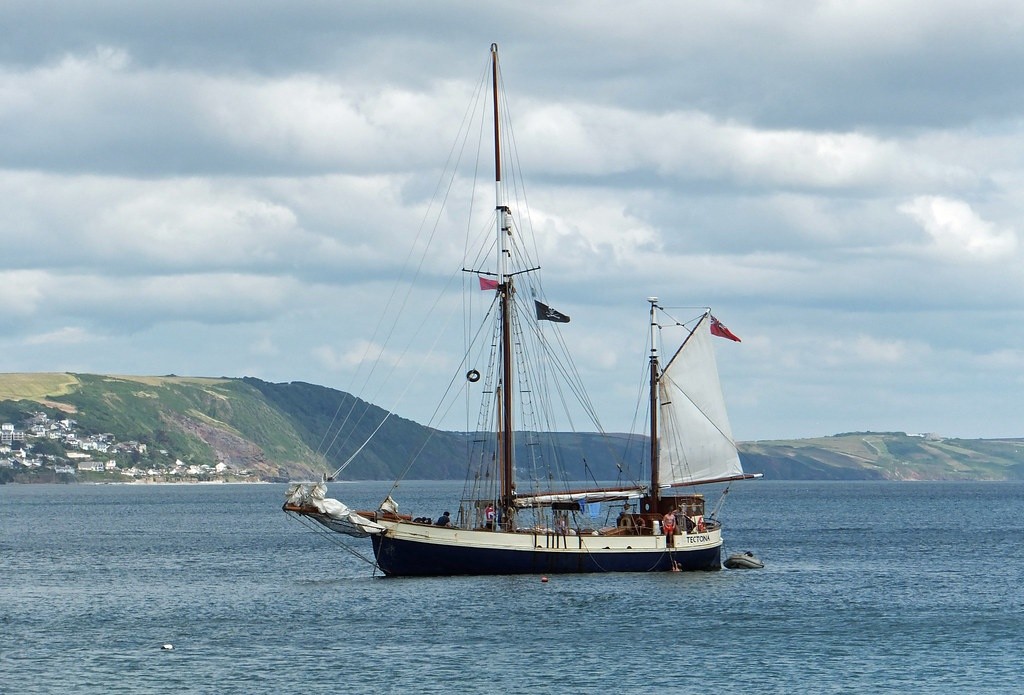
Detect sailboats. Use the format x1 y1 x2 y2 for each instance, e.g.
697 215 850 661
282 44 765 577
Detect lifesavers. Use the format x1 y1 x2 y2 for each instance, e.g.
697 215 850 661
636 518 645 526
621 517 631 526
698 517 706 533
663 514 675 531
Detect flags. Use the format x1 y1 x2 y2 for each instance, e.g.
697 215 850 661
534 300 570 323
711 315 741 343
479 277 499 290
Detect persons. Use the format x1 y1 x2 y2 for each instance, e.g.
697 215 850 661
437 512 452 527
663 510 676 547
486 503 497 530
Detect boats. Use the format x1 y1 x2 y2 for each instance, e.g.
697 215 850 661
725 551 763 571
